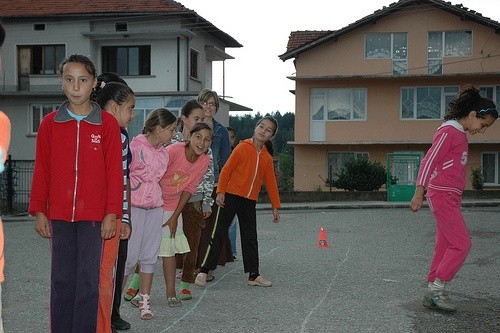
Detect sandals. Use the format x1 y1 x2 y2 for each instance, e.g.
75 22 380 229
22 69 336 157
139 293 154 320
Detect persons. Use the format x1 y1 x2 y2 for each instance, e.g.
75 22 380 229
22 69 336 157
90 70 238 333
194 115 282 288
0 22 12 333
27 53 124 333
408 85 498 312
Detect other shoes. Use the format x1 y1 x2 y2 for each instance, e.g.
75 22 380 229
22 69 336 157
423 289 461 312
111 318 131 333
248 275 272 286
195 272 207 287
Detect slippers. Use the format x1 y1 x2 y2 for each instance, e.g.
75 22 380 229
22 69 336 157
180 289 192 301
124 288 139 301
130 295 141 308
167 297 179 306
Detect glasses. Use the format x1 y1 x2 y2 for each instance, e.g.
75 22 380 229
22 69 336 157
202 101 216 106
